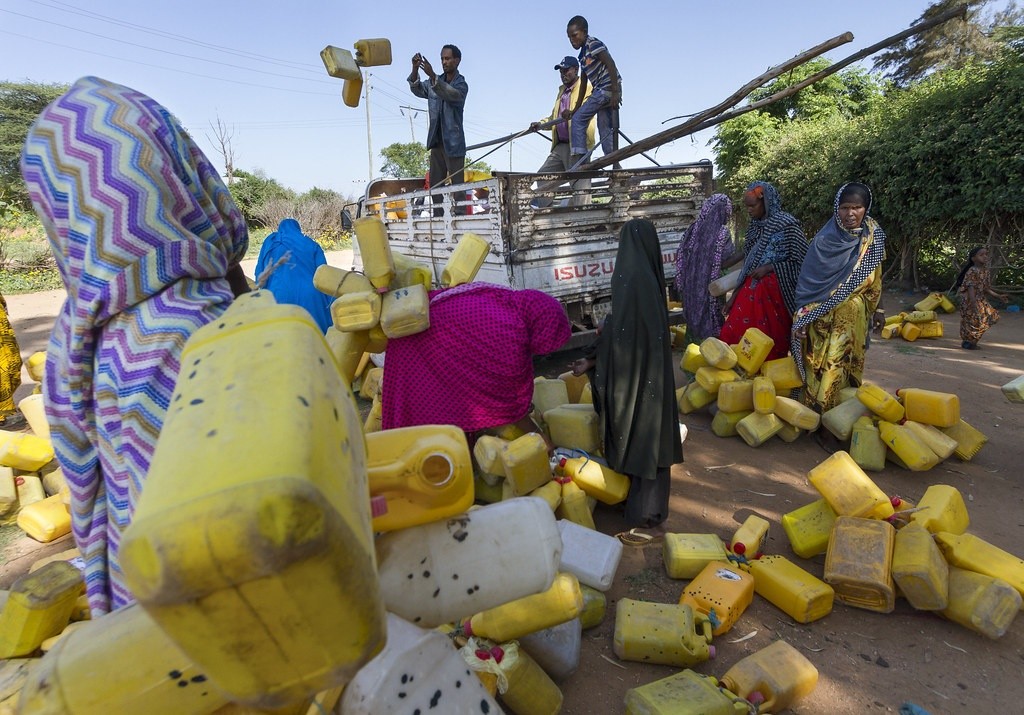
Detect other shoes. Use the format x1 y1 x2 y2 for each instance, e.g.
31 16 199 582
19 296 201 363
605 178 613 185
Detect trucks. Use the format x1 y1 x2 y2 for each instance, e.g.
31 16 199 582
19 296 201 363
340 158 713 353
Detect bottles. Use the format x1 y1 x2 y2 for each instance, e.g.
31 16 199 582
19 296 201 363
0 214 1024 715
341 78 363 108
354 37 392 67
320 44 361 81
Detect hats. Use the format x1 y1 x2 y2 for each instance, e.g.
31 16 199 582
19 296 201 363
554 56 579 70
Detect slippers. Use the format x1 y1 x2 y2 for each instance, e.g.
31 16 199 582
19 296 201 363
620 527 665 546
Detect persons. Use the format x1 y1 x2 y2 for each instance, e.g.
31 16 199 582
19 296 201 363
674 194 745 346
407 44 468 216
531 56 595 210
568 220 685 546
21 76 250 620
719 183 809 362
254 219 335 336
381 282 573 503
566 15 622 185
957 247 1008 350
791 182 887 436
